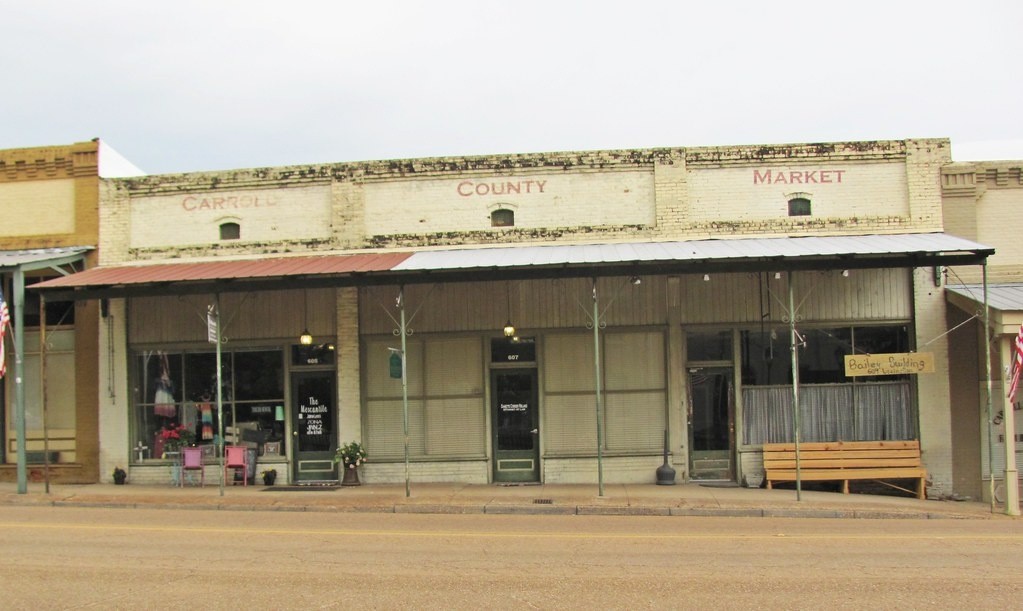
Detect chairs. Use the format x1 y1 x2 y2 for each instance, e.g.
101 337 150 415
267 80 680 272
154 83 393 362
225 446 247 487
180 446 205 488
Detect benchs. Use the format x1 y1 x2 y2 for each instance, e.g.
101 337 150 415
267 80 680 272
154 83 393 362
762 440 926 500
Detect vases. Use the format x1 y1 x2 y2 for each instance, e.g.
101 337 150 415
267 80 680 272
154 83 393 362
341 468 361 486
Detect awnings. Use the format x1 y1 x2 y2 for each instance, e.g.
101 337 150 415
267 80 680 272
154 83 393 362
28 228 997 289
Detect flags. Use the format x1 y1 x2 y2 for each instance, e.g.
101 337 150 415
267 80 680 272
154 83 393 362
0 283 10 379
1007 322 1023 404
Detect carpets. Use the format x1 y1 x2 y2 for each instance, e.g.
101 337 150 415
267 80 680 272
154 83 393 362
259 487 345 491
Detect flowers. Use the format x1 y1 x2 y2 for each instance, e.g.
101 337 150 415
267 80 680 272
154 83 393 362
332 440 368 469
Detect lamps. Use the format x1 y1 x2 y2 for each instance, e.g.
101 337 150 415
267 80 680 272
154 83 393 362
503 280 515 336
300 285 312 345
